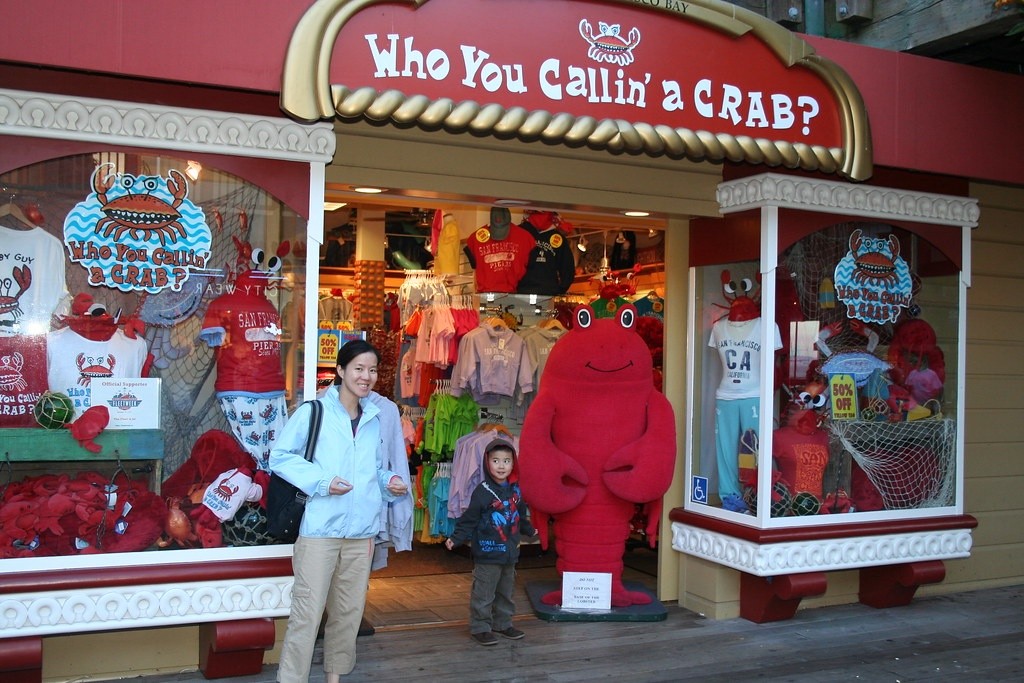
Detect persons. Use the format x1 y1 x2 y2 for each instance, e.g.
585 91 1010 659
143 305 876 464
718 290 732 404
276 340 408 683
200 269 288 475
707 296 784 503
445 439 539 646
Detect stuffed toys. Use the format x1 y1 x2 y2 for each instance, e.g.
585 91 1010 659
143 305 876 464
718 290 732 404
0 405 269 559
517 303 678 608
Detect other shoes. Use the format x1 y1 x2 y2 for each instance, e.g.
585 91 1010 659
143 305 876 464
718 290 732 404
470 632 499 646
491 626 526 640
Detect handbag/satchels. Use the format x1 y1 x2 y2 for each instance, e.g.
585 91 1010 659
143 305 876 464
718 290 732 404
268 400 322 543
